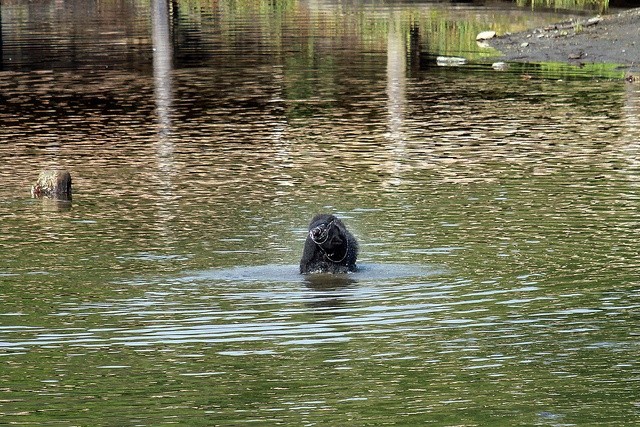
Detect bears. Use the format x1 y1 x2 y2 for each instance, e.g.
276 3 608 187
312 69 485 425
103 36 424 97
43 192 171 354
299 214 360 275
